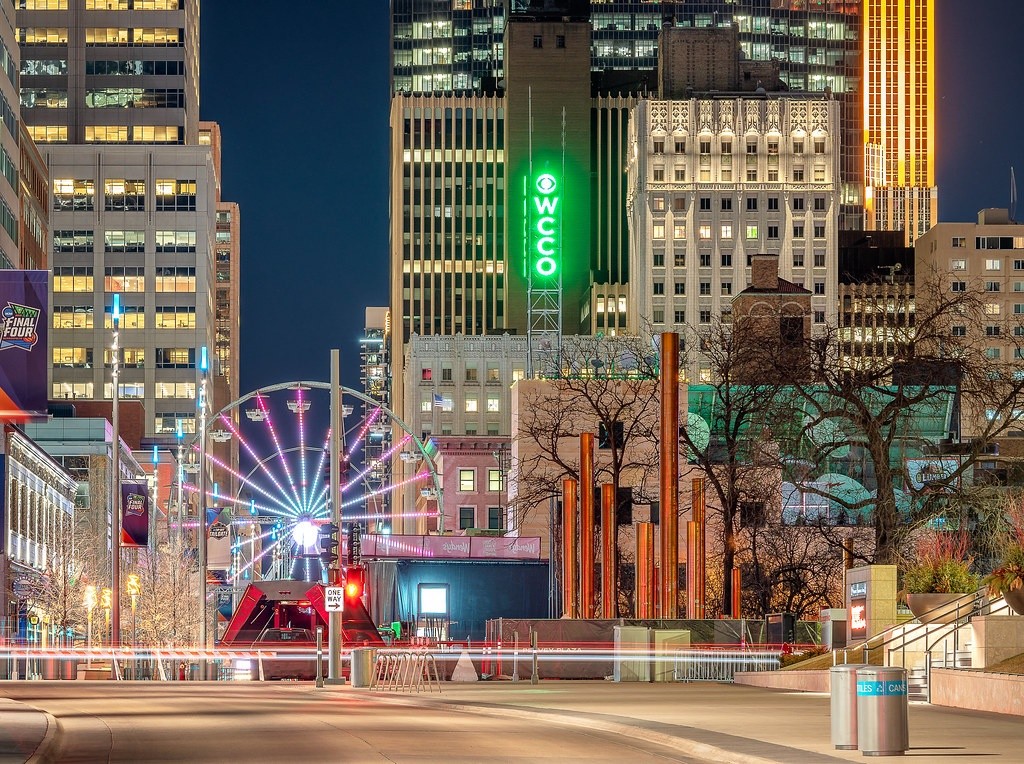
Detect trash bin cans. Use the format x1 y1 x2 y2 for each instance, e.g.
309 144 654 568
857 666 909 757
350 648 377 688
42 658 59 680
61 660 78 680
829 663 870 750
